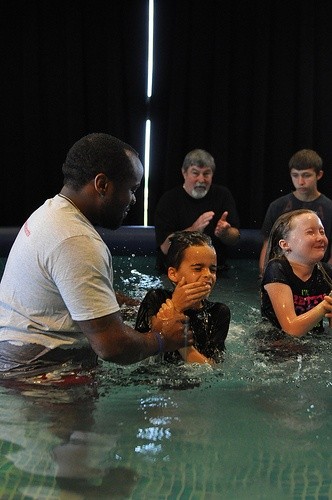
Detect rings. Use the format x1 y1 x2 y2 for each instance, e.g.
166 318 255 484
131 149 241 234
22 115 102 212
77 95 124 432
185 315 188 324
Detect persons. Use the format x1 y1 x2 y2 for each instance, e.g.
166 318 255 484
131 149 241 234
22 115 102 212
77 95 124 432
253 209 332 363
6 430 138 499
0 133 195 493
131 230 230 391
154 149 241 280
259 150 332 281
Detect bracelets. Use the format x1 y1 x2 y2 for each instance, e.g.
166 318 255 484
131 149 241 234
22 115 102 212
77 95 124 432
155 332 162 353
